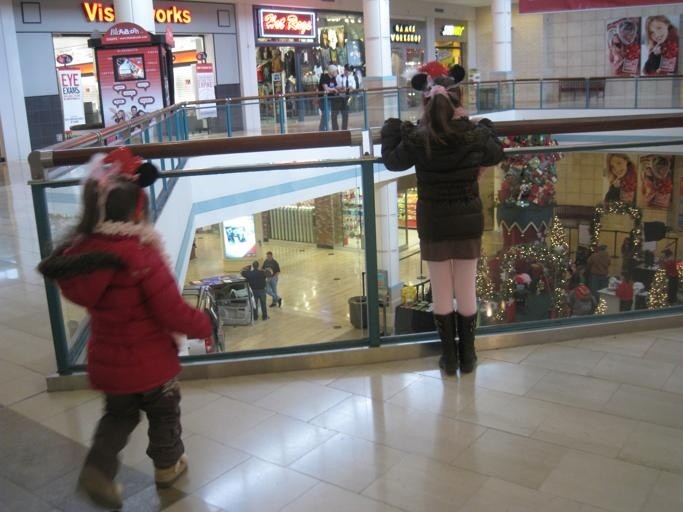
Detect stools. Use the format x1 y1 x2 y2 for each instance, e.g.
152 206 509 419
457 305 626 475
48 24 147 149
64 130 72 140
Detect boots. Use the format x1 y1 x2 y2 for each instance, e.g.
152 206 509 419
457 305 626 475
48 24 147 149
432 309 458 374
454 308 479 374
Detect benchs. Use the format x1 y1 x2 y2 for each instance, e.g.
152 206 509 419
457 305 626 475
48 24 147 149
70 123 102 135
559 79 605 101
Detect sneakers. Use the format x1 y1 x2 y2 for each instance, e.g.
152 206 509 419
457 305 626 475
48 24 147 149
253 314 271 321
78 463 124 512
277 297 282 308
269 303 276 308
152 453 187 488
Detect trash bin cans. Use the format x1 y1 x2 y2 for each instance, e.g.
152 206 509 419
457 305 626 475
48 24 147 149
477 88 496 110
349 296 370 329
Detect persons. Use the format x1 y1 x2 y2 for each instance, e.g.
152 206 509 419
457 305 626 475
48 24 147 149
504 236 678 325
642 15 678 76
240 260 271 320
380 63 505 374
260 252 282 308
108 104 152 145
330 63 356 131
604 154 637 204
318 64 341 130
35 150 220 511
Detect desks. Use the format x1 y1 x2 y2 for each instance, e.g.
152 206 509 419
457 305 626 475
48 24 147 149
597 287 640 314
403 276 431 301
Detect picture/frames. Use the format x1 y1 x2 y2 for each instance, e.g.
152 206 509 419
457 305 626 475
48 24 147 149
218 10 230 27
22 2 41 23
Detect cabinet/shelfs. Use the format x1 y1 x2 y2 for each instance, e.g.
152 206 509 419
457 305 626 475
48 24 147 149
398 187 418 229
